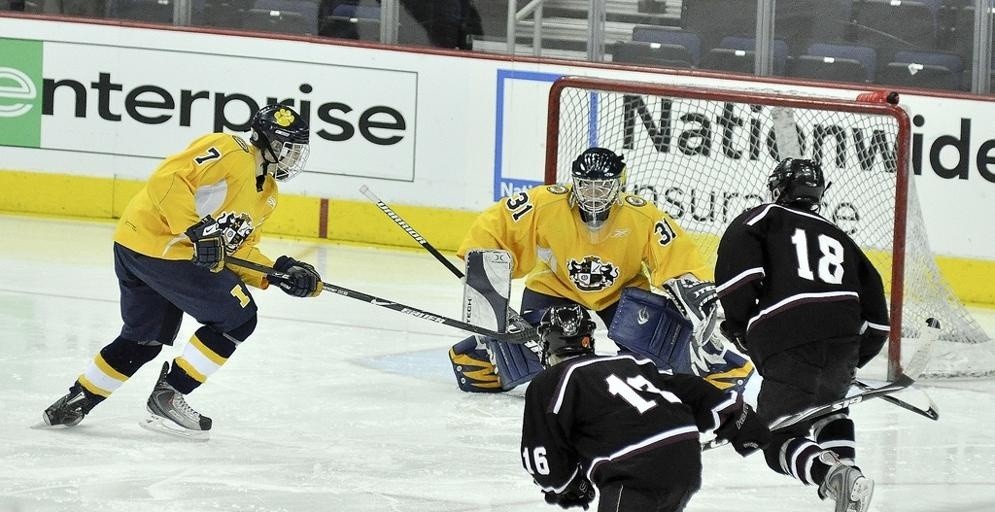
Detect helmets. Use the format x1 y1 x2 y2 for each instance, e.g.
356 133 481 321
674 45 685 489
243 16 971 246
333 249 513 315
572 148 628 213
768 158 826 212
537 304 597 366
250 104 310 183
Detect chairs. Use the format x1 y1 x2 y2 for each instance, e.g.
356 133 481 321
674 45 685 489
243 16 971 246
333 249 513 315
612 0 964 92
240 0 404 44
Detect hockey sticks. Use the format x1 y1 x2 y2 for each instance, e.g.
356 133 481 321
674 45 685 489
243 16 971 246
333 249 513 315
851 379 939 421
224 257 540 343
701 318 939 452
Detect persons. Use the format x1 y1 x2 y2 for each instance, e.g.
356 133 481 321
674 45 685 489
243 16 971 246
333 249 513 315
43 103 324 432
713 158 891 512
445 147 754 394
520 305 772 512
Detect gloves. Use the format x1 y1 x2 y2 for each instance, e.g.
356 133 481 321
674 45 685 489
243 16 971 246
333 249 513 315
545 467 595 508
723 406 773 457
185 216 227 274
267 256 322 297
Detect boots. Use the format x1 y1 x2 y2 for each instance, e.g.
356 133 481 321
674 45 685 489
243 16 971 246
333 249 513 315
148 361 211 430
46 387 89 426
817 449 865 512
